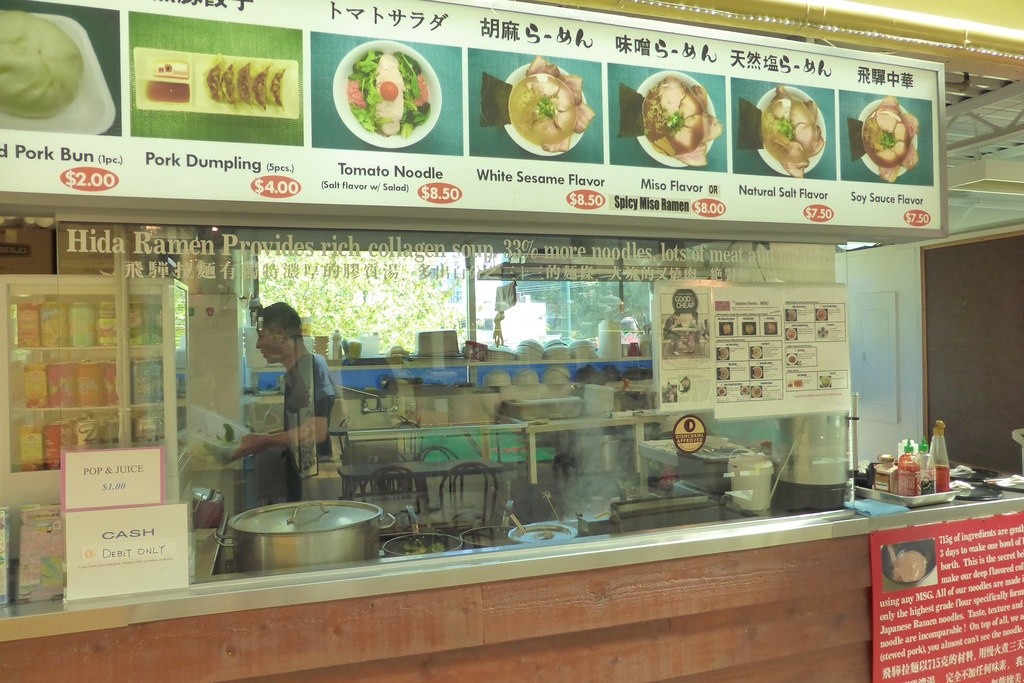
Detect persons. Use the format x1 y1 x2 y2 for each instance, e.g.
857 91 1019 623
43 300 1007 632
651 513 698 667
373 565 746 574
229 303 347 503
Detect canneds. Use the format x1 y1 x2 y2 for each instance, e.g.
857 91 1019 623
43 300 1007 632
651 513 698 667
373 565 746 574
39 300 162 349
42 412 163 470
46 359 163 407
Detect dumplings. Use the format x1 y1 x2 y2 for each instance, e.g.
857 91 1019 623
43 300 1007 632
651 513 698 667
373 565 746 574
203 54 286 111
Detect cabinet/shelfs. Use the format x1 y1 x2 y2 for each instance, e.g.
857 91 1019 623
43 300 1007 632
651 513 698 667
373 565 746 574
0 273 192 560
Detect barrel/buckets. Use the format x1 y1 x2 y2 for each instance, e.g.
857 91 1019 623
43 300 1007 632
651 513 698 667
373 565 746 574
580 434 620 473
772 411 850 511
727 448 775 512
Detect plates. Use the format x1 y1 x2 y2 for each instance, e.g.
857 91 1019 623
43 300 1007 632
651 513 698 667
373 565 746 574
949 480 1003 500
982 480 1024 492
950 465 1002 482
132 45 300 121
0 9 116 135
483 344 515 361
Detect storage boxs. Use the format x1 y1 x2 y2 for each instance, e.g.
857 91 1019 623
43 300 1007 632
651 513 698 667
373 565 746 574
0 227 56 275
499 397 585 420
404 432 522 462
520 441 555 463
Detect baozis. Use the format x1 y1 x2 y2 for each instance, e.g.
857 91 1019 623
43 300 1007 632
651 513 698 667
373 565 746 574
0 10 83 117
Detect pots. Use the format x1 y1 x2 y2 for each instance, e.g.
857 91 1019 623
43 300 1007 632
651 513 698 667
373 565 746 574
460 498 517 547
207 497 397 574
380 503 462 556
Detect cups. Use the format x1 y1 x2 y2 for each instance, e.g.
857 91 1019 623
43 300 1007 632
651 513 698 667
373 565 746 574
638 334 652 357
622 342 640 356
342 338 362 359
299 315 316 337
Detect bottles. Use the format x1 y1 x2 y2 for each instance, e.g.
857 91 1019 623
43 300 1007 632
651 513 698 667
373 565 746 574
597 318 622 359
41 299 95 347
357 331 380 358
328 329 342 360
873 419 951 495
619 378 630 394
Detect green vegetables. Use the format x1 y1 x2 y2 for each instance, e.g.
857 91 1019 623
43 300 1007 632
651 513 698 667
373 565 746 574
347 50 431 138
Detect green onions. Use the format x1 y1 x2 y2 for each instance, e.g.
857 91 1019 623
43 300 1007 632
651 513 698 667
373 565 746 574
776 117 797 141
534 96 555 122
664 110 685 134
881 132 896 149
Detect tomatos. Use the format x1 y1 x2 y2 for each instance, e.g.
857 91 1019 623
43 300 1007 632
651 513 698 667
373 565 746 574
380 81 398 101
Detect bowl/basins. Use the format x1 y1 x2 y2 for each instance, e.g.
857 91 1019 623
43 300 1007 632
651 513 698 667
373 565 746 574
613 390 639 410
417 330 464 356
483 365 572 386
583 382 616 415
503 63 588 155
463 340 488 361
331 40 443 150
185 401 253 467
635 70 717 168
507 521 579 545
856 100 919 177
755 86 828 176
574 363 646 384
515 339 595 360
